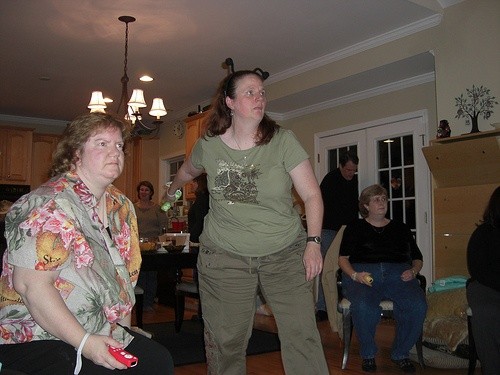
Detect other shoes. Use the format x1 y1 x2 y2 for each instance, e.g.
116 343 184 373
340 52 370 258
392 358 416 373
191 315 204 322
316 310 328 320
362 358 377 373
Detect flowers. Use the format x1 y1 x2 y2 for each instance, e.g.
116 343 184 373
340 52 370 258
454 83 498 119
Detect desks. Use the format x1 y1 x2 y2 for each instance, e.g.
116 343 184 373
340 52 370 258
133 242 202 328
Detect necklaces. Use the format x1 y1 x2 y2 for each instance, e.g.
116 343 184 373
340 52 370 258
233 135 247 159
372 219 386 233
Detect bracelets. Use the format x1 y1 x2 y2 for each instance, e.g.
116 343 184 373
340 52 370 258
166 188 174 198
74 334 91 375
412 269 415 276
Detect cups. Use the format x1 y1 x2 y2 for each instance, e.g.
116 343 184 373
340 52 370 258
176 234 190 250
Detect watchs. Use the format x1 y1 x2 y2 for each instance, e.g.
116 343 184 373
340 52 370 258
307 236 321 243
351 272 356 281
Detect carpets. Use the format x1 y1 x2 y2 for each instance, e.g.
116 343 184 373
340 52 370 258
134 318 280 366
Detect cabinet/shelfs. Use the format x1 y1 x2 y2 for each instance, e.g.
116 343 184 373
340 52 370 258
0 123 34 184
112 134 161 203
30 134 64 192
182 112 210 201
422 129 500 287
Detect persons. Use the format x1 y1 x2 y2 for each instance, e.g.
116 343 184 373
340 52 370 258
316 152 359 322
188 173 209 319
161 70 331 374
132 181 168 311
338 185 427 372
0 112 174 375
466 185 500 288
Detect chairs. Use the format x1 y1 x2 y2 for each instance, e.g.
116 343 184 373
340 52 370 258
421 287 481 375
336 263 431 372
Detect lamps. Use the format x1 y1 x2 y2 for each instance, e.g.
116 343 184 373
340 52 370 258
88 16 167 124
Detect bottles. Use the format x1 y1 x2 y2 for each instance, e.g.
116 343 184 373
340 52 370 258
166 234 177 246
159 228 170 242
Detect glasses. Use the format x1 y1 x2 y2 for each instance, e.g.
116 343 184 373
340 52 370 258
376 197 386 203
347 168 358 172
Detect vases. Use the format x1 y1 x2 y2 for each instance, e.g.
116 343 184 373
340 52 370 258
471 117 481 134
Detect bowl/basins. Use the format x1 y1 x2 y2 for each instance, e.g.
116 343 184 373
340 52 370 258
155 242 172 252
140 243 155 251
162 245 187 253
